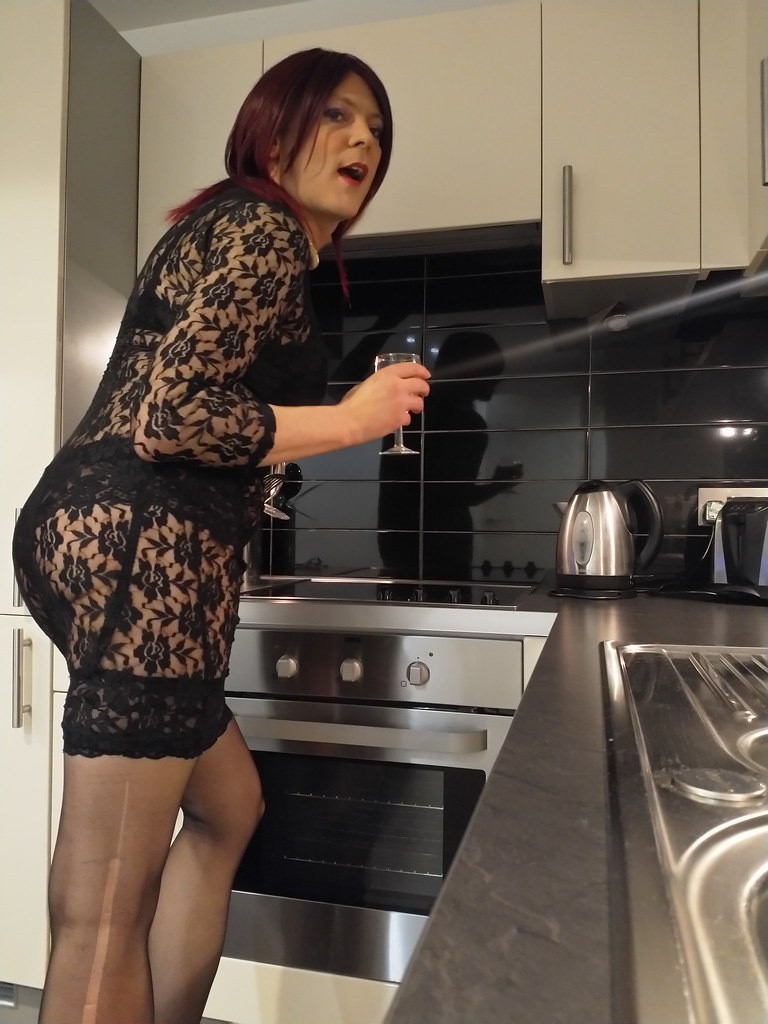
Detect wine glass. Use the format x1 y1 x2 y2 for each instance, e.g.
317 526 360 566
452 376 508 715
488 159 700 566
371 353 424 455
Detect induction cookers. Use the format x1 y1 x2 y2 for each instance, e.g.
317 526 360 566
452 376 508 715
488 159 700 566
236 557 539 609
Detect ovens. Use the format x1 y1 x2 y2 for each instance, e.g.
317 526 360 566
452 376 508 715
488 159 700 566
51 626 524 983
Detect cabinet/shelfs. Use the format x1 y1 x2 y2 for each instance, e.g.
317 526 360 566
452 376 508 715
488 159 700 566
136 0 541 276
541 0 768 318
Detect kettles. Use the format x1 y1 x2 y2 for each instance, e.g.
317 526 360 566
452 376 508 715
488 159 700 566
552 478 665 599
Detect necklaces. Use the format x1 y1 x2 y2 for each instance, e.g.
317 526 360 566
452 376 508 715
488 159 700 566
305 233 319 271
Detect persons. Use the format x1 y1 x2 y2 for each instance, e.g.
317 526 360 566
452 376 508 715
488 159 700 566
12 47 432 1024
